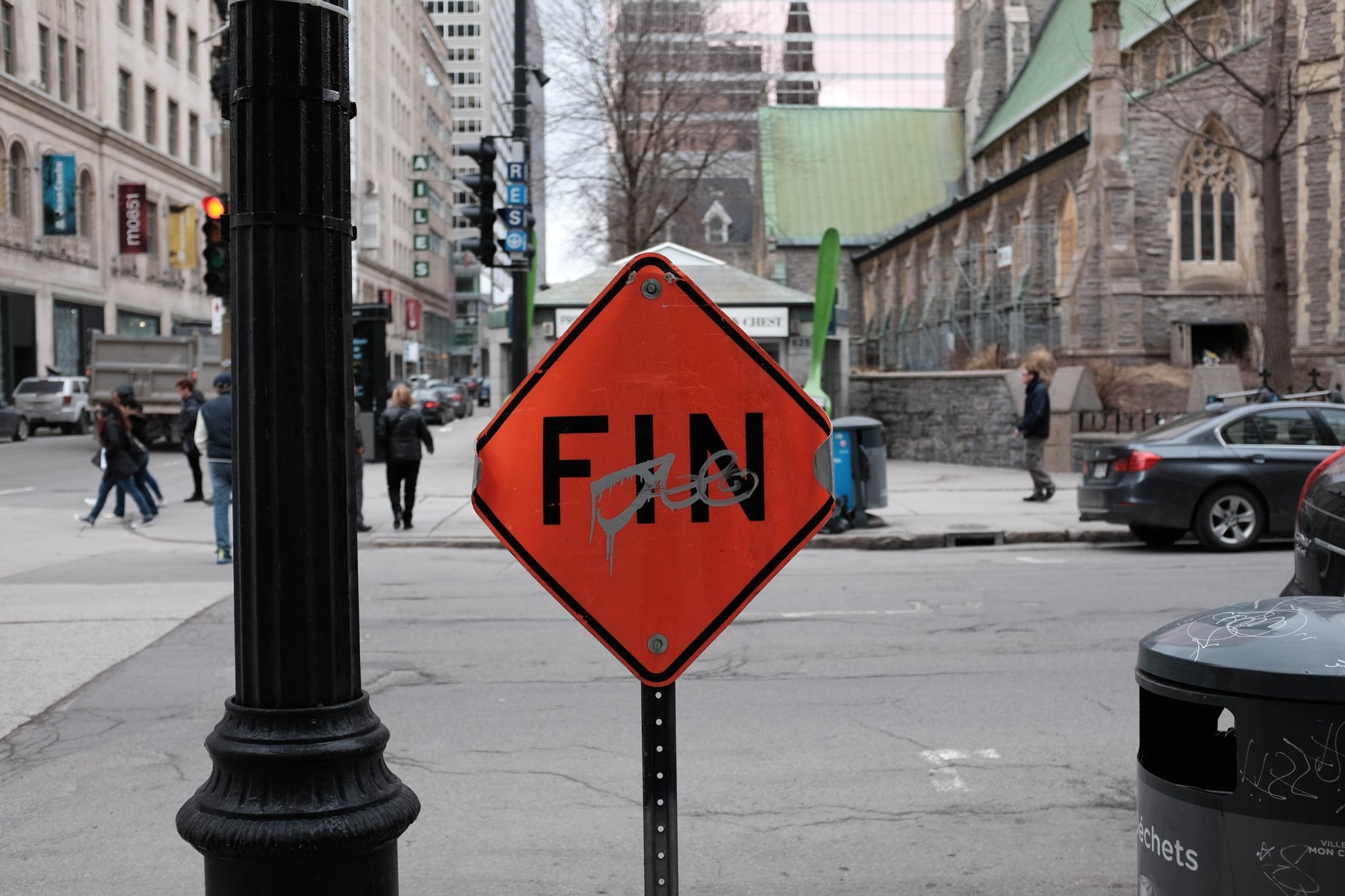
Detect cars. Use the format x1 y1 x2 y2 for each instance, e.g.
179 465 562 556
403 371 491 427
1278 443 1345 597
1077 400 1345 553
0 394 29 441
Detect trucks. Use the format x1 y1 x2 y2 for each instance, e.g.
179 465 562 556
89 329 200 450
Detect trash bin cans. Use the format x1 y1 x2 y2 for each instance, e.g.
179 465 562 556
1133 595 1345 896
831 415 888 528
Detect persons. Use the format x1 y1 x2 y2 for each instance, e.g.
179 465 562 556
175 378 207 503
378 384 434 530
72 384 164 527
1014 366 1056 502
194 372 233 562
354 386 371 533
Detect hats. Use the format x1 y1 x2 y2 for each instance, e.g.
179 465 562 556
213 371 231 388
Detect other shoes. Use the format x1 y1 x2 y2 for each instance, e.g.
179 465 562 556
404 522 412 529
83 498 98 507
74 515 94 527
217 549 232 564
130 517 154 528
1022 494 1044 502
394 513 402 529
357 526 372 532
1045 485 1057 501
184 494 204 501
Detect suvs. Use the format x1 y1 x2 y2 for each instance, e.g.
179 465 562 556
13 375 94 435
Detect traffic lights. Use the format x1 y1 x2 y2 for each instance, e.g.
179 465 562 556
201 192 230 296
453 136 497 266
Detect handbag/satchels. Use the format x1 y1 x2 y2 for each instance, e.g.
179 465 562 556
127 434 147 456
